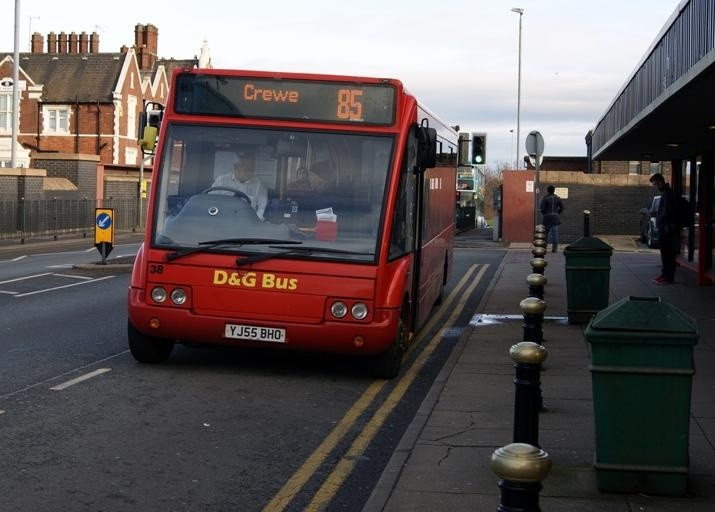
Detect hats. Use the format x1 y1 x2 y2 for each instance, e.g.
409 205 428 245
310 159 336 182
547 185 554 193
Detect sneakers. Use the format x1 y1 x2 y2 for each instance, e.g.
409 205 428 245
653 273 674 284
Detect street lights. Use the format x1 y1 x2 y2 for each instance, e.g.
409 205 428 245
509 128 515 171
509 5 523 173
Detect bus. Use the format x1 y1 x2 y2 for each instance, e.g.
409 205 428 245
451 158 486 229
126 65 462 370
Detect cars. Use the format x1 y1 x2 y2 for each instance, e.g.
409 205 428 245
635 192 700 248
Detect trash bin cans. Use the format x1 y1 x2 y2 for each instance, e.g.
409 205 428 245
585 294 700 494
563 237 614 323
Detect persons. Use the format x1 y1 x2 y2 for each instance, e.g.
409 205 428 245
289 168 311 192
208 151 267 221
540 185 563 253
650 173 694 284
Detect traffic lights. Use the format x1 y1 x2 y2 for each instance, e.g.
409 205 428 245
472 132 488 164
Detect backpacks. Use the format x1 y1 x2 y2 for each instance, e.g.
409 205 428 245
667 192 692 226
